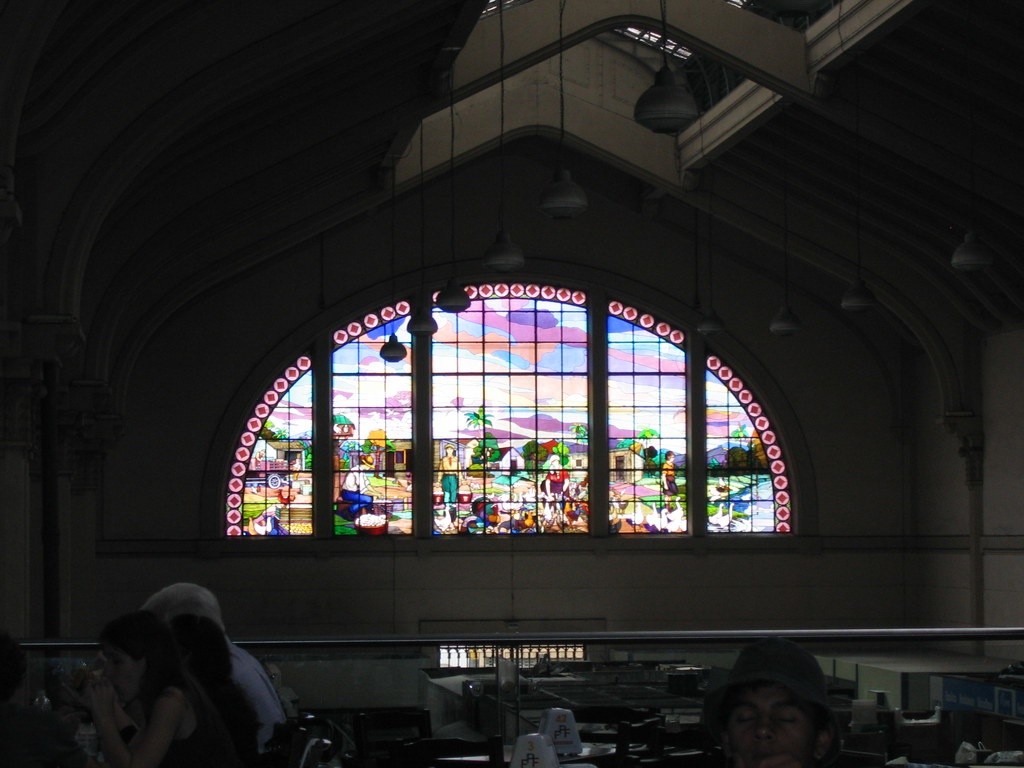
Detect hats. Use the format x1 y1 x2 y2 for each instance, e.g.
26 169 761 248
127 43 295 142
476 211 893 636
700 638 839 768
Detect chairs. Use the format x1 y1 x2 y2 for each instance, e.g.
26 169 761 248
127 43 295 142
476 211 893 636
612 713 667 768
877 708 953 768
651 726 728 768
392 734 506 768
274 718 332 768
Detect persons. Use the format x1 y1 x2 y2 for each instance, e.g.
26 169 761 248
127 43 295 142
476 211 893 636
89 583 298 768
704 638 841 768
0 632 94 768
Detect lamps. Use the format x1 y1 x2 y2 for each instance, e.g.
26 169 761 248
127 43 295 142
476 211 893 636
634 1 698 135
405 0 439 340
536 1 587 222
481 1 525 277
842 0 883 316
697 2 725 338
379 1 407 364
768 0 806 338
434 1 471 314
951 0 994 274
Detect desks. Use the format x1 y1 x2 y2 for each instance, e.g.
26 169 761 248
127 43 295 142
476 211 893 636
436 743 617 768
